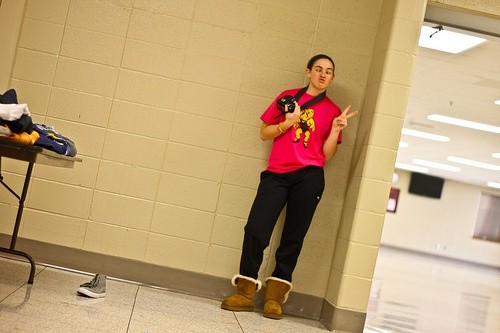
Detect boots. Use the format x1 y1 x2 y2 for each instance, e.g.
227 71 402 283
263 276 293 319
221 274 262 311
77 274 107 299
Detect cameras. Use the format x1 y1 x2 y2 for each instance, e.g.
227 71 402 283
277 95 294 113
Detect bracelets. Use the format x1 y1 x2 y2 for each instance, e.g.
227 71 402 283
277 124 285 134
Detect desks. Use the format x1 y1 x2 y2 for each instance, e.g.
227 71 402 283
0 135 83 283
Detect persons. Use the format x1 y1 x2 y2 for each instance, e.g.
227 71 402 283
220 54 359 321
